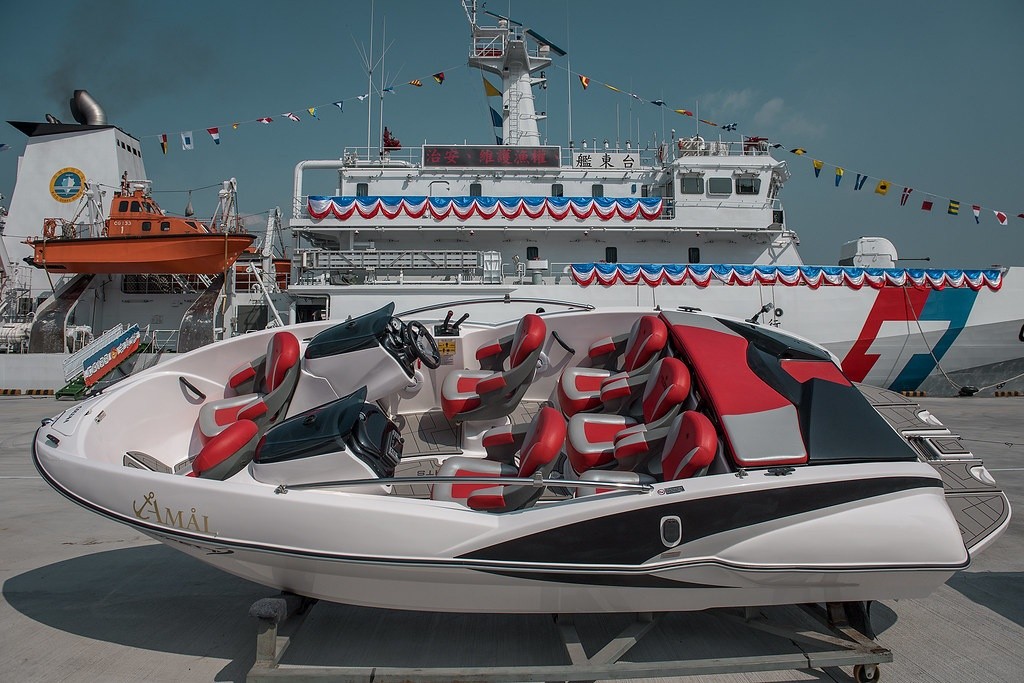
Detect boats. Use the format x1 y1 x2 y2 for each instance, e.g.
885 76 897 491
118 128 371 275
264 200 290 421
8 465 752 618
17 170 258 277
0 1 1024 396
31 296 1014 617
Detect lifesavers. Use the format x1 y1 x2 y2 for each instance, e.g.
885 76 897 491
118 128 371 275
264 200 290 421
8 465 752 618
27 236 31 242
44 219 56 238
34 236 38 241
65 223 76 239
102 227 109 236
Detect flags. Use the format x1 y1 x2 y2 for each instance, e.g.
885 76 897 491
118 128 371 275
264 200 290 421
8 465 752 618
207 128 219 145
652 99 667 108
790 148 807 155
972 205 980 224
947 199 960 215
771 143 785 149
854 173 868 190
900 187 913 206
920 193 937 211
484 78 503 97
993 210 1008 226
674 110 693 116
874 180 892 195
158 134 168 154
490 106 503 127
497 136 503 145
181 131 193 151
1017 213 1024 219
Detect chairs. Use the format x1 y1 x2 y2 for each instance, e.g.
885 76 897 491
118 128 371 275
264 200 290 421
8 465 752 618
439 313 546 423
197 332 302 446
578 410 718 497
564 356 693 474
558 315 669 416
430 407 568 513
